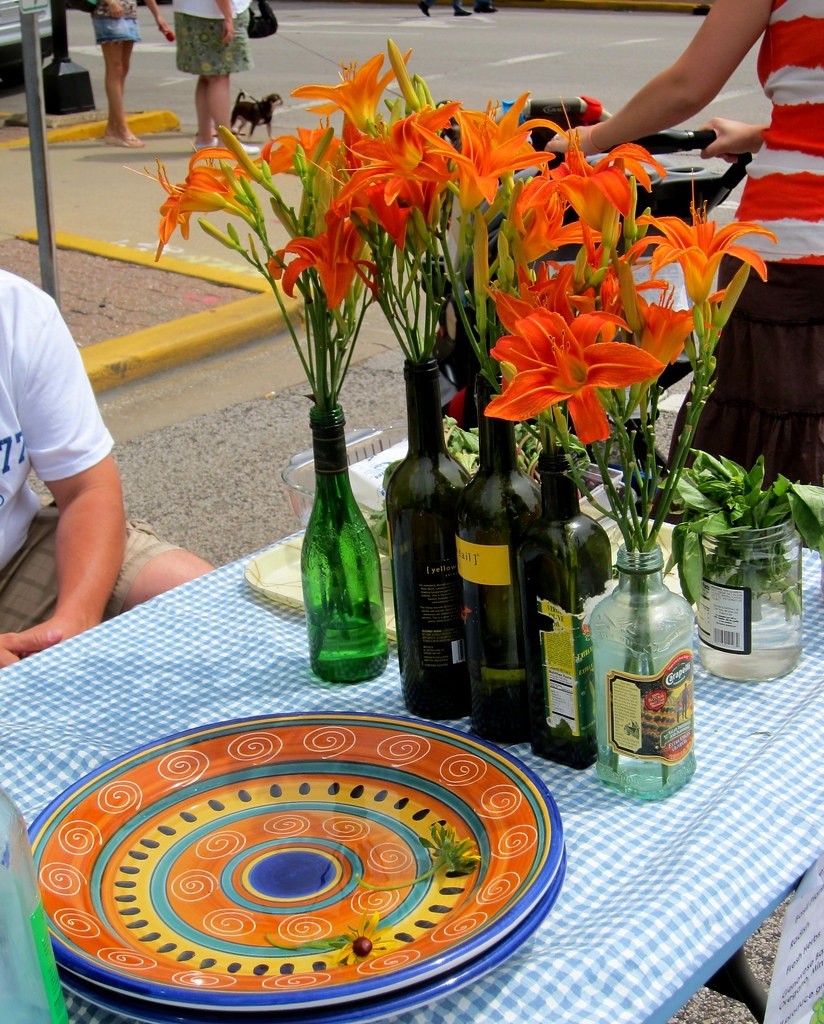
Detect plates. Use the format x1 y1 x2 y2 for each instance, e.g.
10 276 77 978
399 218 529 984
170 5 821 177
28 713 566 1024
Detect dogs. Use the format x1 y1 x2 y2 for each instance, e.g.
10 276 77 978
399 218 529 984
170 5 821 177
229 91 283 142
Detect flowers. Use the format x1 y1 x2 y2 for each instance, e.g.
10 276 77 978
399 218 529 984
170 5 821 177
123 56 782 778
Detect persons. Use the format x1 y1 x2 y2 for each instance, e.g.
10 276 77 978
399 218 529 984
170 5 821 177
91 0 167 147
172 0 259 155
0 268 215 668
418 0 497 17
546 1 824 487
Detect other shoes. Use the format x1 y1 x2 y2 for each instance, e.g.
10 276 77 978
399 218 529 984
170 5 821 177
240 142 259 155
194 137 218 150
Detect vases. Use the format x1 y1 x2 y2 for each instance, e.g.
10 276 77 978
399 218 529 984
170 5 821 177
303 358 694 802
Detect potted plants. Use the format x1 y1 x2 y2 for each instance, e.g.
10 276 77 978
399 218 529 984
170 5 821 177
653 444 824 681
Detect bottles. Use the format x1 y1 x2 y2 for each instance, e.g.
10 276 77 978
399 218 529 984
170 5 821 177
0 787 69 1024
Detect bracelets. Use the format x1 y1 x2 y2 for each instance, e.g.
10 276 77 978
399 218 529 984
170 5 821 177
589 122 612 153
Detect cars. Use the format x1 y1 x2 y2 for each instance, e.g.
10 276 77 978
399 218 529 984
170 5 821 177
0 0 53 85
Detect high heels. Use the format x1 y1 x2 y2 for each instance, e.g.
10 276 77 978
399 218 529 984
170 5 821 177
104 133 145 148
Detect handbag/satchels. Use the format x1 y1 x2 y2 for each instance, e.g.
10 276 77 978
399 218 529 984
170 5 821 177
248 0 278 38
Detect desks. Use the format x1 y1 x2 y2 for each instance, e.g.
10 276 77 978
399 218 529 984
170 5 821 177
1 527 824 1024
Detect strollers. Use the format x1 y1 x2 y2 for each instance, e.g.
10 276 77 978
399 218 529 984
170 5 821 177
431 96 753 511
